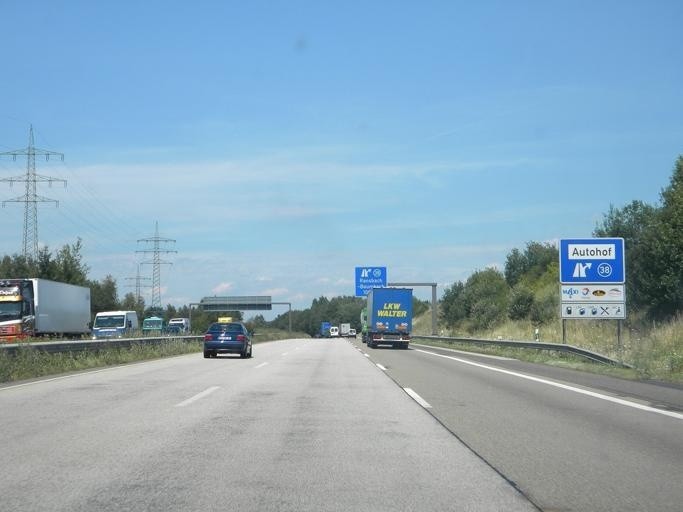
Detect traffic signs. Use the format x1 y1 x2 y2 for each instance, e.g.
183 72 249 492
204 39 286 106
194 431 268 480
355 267 387 297
559 237 626 284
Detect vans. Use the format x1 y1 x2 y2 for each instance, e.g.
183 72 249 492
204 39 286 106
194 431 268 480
347 329 356 339
329 327 339 337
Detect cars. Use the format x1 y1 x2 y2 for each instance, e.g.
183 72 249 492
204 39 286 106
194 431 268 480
166 326 183 335
203 322 252 358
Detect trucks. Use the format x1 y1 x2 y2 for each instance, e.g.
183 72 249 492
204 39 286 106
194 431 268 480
0 277 91 344
168 318 190 334
339 323 351 338
143 316 166 336
360 288 413 349
89 311 139 340
